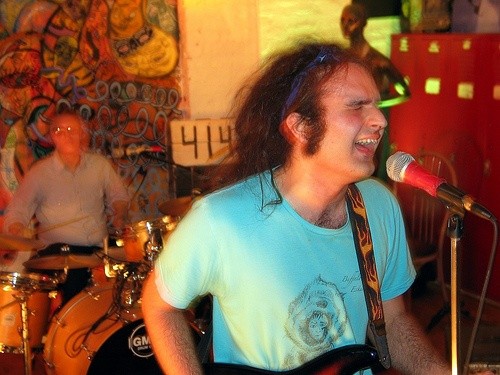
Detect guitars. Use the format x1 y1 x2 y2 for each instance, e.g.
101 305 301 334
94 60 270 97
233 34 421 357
201 344 497 375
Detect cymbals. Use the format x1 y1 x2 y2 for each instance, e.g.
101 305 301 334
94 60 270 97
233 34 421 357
158 196 193 218
22 255 98 269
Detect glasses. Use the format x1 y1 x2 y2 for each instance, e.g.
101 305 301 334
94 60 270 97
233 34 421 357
49 125 78 137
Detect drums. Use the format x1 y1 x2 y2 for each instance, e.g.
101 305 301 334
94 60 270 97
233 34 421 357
122 215 178 263
90 247 130 286
0 270 58 355
42 282 206 375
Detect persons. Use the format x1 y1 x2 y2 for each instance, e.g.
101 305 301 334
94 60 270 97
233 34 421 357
4 111 129 305
141 42 500 375
339 5 411 183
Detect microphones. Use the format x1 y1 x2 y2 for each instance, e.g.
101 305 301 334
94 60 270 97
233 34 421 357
386 151 493 220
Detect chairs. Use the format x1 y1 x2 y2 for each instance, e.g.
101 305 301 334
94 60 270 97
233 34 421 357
392 152 459 305
